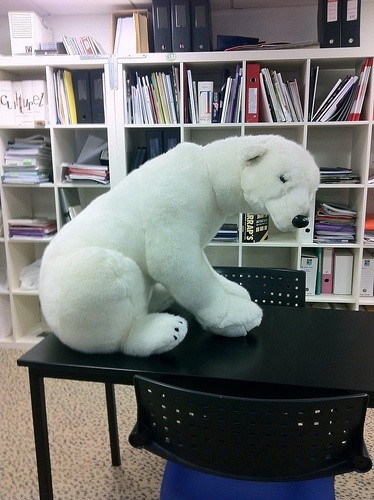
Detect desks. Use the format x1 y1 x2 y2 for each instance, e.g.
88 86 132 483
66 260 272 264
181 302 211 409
16 306 374 500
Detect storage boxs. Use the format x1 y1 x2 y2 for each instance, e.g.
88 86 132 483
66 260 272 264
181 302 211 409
110 8 152 55
300 252 319 296
359 252 374 297
332 250 354 295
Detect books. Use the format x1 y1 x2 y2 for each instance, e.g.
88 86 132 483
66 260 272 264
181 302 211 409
9 217 56 236
213 223 238 242
60 163 109 184
316 166 360 185
1 132 51 184
125 56 374 125
316 202 357 242
61 0 216 55
54 69 104 123
63 204 82 222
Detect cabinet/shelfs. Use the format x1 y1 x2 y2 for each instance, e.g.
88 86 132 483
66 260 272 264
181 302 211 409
0 0 374 350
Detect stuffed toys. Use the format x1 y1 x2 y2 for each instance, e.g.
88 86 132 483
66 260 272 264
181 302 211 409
37 134 321 357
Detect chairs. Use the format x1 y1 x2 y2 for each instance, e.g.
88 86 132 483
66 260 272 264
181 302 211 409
210 266 306 307
128 374 372 500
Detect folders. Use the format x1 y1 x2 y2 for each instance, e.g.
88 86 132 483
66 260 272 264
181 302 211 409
322 247 334 294
320 0 360 48
152 0 211 51
246 63 260 123
